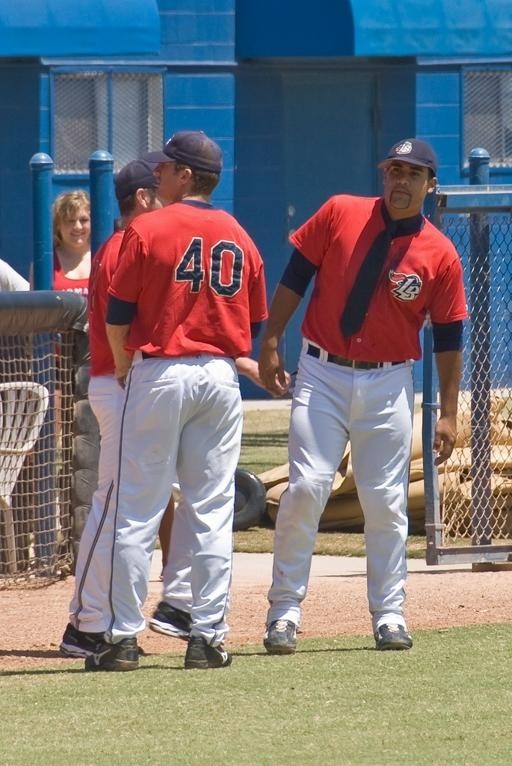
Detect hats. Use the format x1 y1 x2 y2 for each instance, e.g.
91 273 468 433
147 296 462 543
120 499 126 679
142 130 223 174
115 160 157 201
379 138 438 178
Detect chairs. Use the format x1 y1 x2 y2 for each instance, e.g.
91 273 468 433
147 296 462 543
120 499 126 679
1 377 52 577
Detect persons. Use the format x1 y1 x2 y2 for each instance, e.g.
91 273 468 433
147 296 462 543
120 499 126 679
259 135 471 654
0 256 34 585
82 128 270 670
50 188 91 535
57 146 292 659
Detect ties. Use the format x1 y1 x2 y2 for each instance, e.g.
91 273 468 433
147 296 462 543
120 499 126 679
340 203 425 337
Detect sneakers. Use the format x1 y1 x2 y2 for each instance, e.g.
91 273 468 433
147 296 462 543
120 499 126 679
83 638 140 672
263 620 298 654
148 603 194 640
60 623 143 659
184 636 233 670
374 623 413 651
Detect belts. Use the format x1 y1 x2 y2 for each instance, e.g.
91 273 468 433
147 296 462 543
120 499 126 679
307 343 407 370
141 350 157 361
